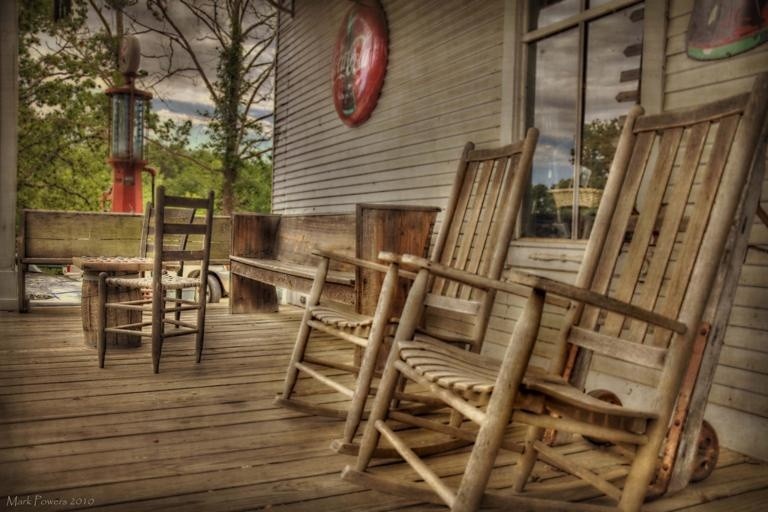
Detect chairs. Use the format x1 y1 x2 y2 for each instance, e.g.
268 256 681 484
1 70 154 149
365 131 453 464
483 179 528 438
98 185 214 375
274 128 540 457
341 74 768 512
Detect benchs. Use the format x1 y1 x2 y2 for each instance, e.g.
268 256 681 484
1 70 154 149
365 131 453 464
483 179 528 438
15 203 443 380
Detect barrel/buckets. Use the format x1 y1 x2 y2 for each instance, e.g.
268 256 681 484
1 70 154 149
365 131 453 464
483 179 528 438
80 270 144 349
